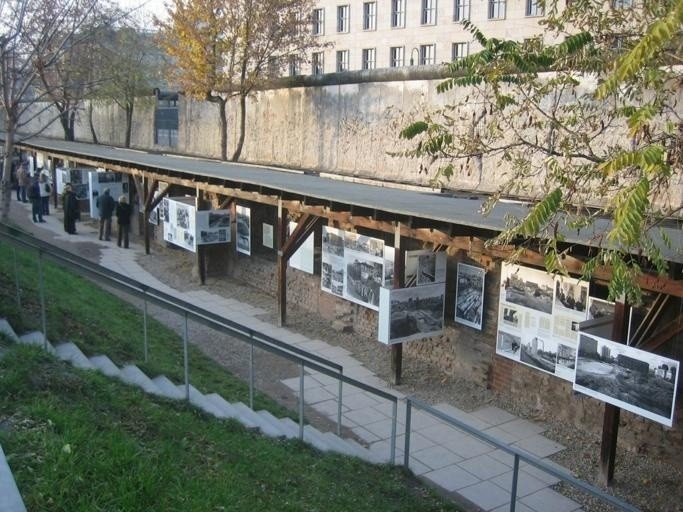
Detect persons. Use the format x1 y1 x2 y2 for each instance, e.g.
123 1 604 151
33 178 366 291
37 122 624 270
0 148 134 251
